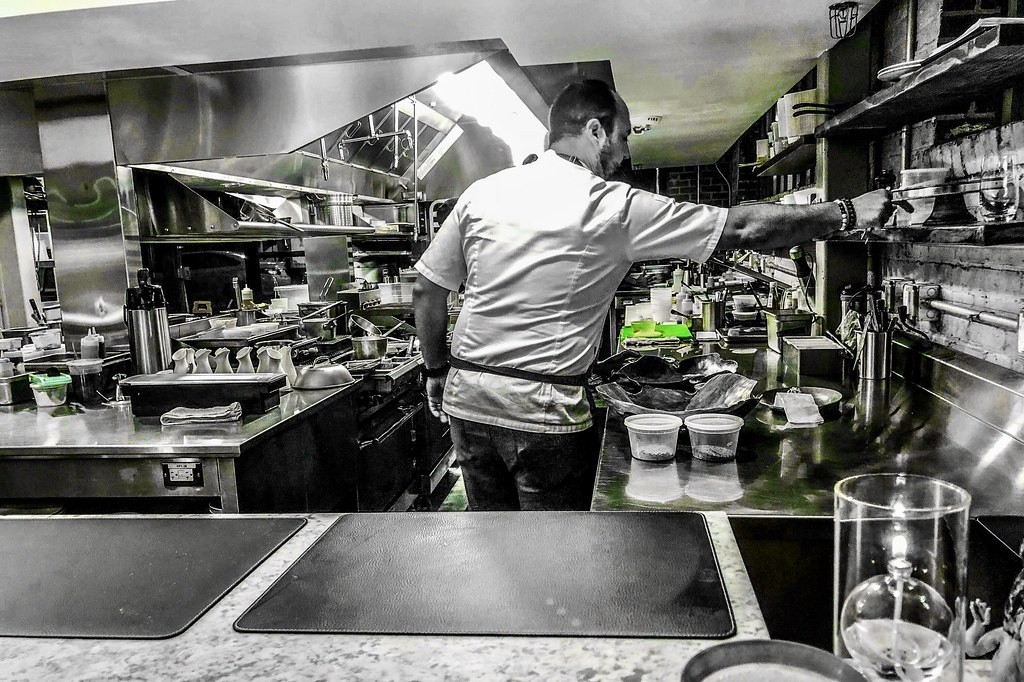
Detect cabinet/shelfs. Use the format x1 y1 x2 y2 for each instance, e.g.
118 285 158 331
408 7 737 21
0 170 424 344
707 0 1024 375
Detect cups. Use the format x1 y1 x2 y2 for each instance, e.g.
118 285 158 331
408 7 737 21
978 155 1019 223
856 331 891 379
832 475 965 682
700 301 727 331
649 287 672 321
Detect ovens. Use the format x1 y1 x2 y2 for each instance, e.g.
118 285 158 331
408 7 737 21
354 380 424 512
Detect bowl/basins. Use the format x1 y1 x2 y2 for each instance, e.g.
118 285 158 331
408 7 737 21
732 295 757 311
632 322 656 332
349 315 381 336
66 359 103 375
685 412 741 459
209 317 237 329
30 329 61 351
352 338 388 358
30 383 67 406
681 641 867 682
622 415 681 461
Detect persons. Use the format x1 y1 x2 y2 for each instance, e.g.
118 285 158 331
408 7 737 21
412 78 894 511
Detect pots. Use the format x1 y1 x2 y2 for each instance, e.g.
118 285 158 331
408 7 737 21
298 302 347 334
303 318 336 339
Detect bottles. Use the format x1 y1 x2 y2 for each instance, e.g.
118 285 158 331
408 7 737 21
241 284 253 310
681 296 693 328
80 327 106 359
677 287 686 323
674 268 683 291
769 281 778 308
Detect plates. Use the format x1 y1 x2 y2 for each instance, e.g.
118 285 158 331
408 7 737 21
762 384 841 408
877 60 923 82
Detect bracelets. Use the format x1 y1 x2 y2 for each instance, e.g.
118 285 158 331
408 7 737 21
834 198 857 233
424 365 450 379
